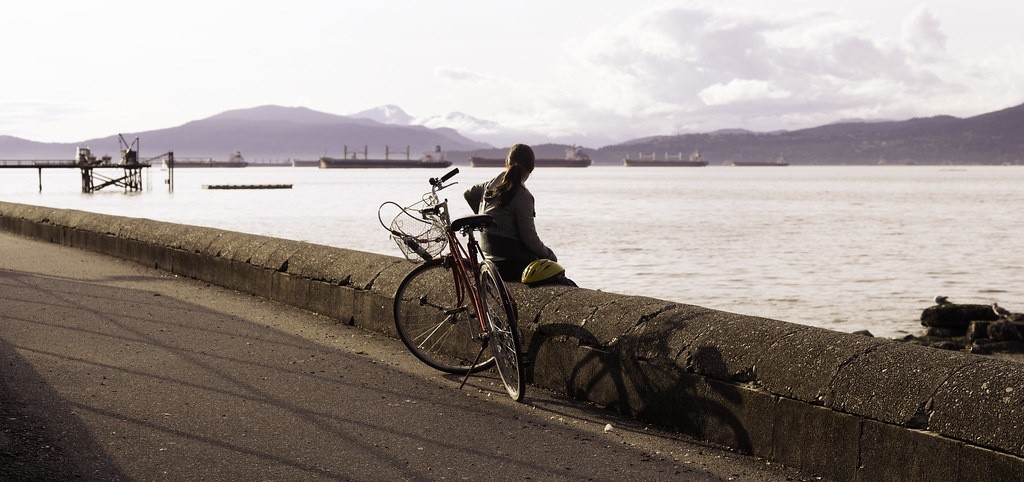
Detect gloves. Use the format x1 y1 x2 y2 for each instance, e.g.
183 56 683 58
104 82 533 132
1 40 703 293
548 249 557 263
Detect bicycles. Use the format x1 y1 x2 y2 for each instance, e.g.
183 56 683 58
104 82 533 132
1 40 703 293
376 166 532 402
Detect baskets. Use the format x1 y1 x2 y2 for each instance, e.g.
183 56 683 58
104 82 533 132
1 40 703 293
390 198 448 263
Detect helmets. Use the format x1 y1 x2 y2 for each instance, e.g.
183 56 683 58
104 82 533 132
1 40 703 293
521 259 565 285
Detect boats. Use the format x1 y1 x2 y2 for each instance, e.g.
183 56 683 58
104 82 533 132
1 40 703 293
319 143 453 169
163 150 249 168
732 161 790 167
467 143 593 168
622 153 709 167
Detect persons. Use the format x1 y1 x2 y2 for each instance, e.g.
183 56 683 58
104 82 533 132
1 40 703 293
463 143 578 288
